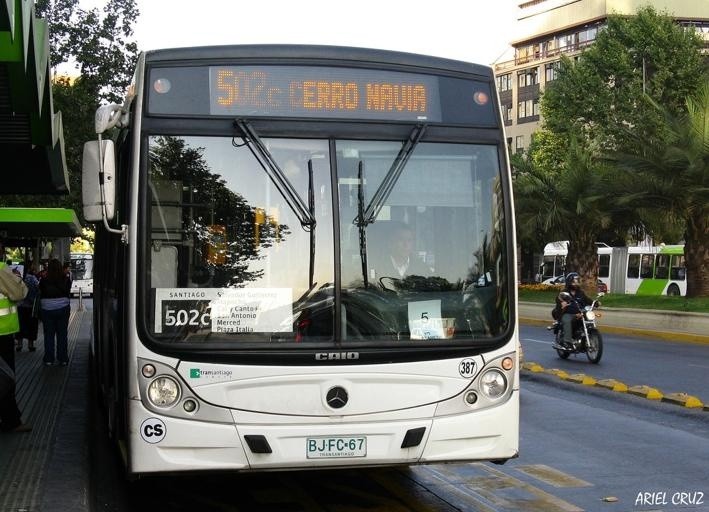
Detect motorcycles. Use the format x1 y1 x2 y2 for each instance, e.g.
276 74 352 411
548 289 605 365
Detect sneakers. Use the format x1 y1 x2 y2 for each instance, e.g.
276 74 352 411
45 358 67 367
12 423 33 432
561 343 573 351
16 344 36 353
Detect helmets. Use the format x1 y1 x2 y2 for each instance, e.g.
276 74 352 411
565 272 581 290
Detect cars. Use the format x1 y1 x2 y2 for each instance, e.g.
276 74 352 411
549 274 608 294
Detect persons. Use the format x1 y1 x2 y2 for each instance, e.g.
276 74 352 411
555 271 602 349
372 223 433 278
0 258 73 433
534 272 541 285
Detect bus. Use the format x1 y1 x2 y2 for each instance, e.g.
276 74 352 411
68 252 95 297
68 40 525 481
538 239 687 296
